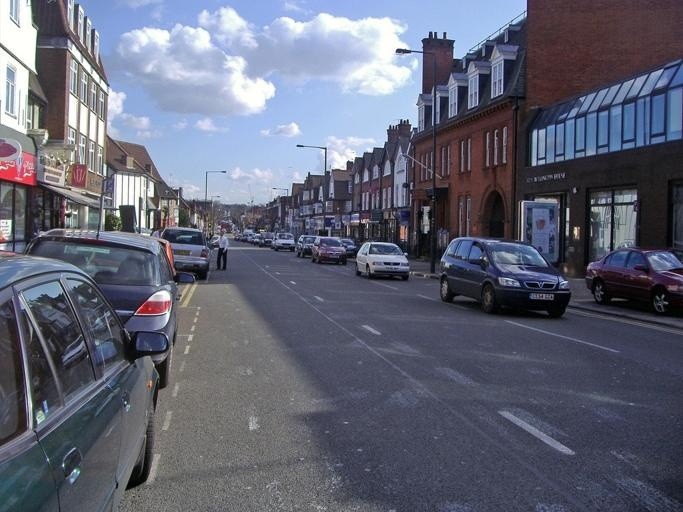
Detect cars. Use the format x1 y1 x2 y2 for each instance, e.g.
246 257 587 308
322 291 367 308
23 230 197 390
341 239 356 256
310 236 346 265
0 250 169 511
354 242 409 281
234 231 295 251
91 231 179 287
583 248 683 316
209 235 220 246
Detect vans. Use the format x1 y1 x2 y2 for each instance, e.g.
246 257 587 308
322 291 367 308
438 235 572 321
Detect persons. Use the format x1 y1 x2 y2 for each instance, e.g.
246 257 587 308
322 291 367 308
208 233 213 241
212 231 229 271
306 232 308 235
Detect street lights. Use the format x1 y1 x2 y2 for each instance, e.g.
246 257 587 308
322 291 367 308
204 170 226 223
295 144 328 235
271 187 288 224
394 47 438 273
210 195 220 234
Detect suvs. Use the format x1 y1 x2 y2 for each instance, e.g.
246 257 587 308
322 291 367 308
295 235 317 257
160 226 212 281
